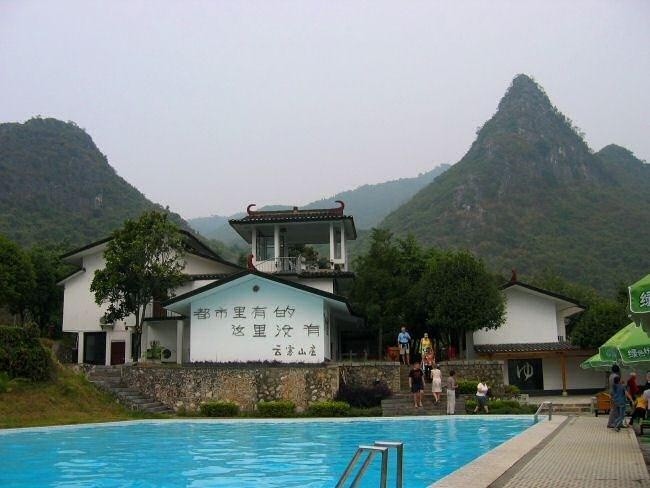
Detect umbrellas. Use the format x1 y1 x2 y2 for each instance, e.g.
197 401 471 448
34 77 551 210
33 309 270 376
579 273 650 371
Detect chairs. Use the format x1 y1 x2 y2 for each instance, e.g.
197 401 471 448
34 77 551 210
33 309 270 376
288 255 320 272
592 392 611 417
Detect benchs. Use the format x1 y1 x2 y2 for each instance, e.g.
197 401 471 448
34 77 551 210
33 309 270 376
639 419 650 435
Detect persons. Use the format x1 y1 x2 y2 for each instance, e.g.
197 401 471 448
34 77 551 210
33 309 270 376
418 332 433 371
607 364 650 432
444 370 458 415
424 347 435 383
409 362 426 408
473 378 491 414
398 325 411 366
430 363 442 405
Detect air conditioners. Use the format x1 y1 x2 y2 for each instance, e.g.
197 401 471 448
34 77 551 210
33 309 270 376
161 346 176 362
